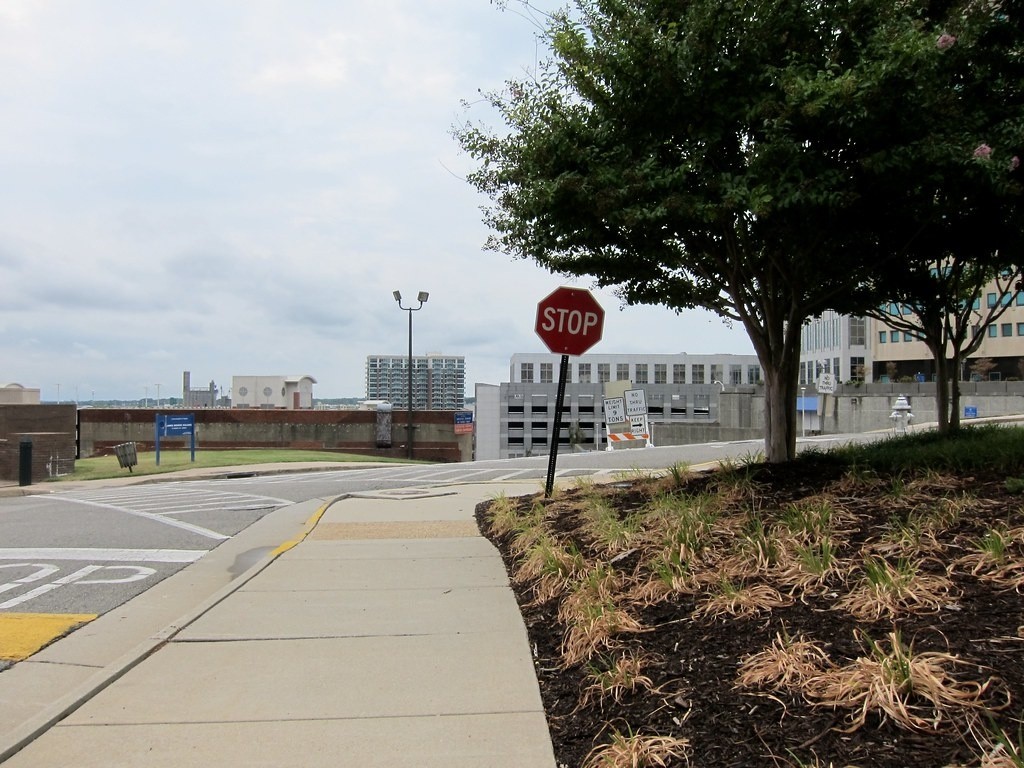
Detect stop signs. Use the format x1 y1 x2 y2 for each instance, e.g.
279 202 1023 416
534 287 606 357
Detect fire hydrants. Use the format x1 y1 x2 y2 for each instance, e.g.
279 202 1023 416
890 393 915 434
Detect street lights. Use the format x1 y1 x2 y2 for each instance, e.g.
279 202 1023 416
393 290 429 458
960 360 965 381
55 383 61 404
155 384 163 407
801 387 806 437
92 389 95 406
